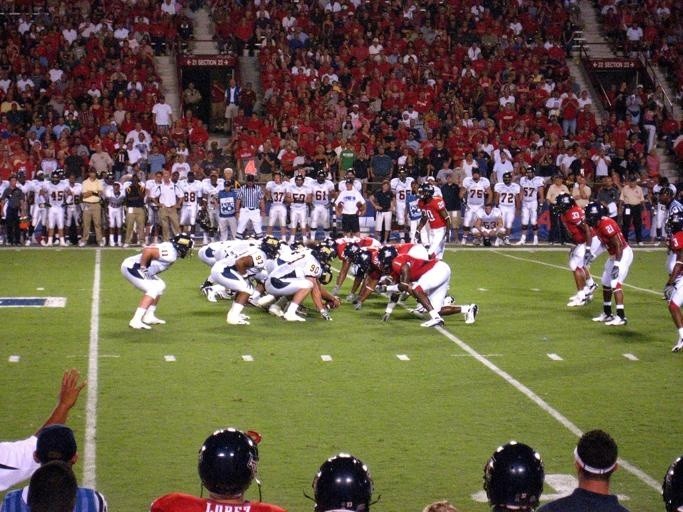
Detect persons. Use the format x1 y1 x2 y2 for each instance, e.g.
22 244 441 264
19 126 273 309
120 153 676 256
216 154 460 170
120 230 478 330
28 460 76 510
1 367 86 490
151 430 283 512
1 425 107 510
553 193 683 352
1 0 682 249
538 429 625 511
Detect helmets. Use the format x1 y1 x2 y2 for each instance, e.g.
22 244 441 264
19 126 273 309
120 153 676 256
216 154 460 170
8 166 116 182
260 234 399 272
667 211 683 231
502 166 537 177
173 231 193 251
417 181 435 200
196 426 264 496
397 166 407 173
223 167 356 187
583 201 606 224
554 193 575 213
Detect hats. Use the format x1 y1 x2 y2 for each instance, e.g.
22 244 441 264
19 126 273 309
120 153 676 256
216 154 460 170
629 176 637 182
655 187 674 195
470 165 481 174
35 423 79 466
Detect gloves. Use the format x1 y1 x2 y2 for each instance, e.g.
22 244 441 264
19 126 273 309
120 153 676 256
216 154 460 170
374 284 387 293
583 249 593 265
380 311 392 322
662 283 676 301
611 265 620 280
317 285 363 322
140 266 155 282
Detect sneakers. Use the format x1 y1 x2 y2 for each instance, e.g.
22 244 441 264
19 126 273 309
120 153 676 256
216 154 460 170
446 236 569 248
420 318 445 328
5 237 160 247
566 281 598 307
201 285 307 326
465 304 479 326
592 312 613 322
604 314 628 326
653 240 662 248
670 334 683 353
126 318 152 331
636 240 645 247
141 315 167 325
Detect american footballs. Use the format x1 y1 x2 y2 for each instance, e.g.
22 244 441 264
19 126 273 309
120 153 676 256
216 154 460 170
325 296 340 309
